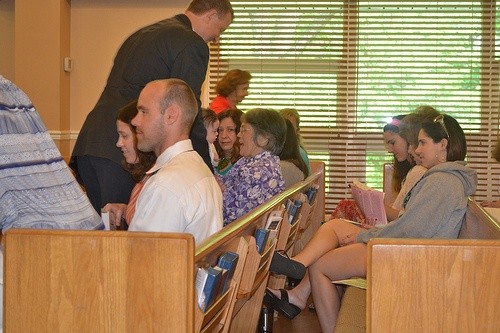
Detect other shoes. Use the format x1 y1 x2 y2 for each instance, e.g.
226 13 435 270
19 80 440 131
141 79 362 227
308 304 315 310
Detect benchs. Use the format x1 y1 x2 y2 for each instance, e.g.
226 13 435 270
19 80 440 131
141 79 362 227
3 160 325 333
332 163 500 333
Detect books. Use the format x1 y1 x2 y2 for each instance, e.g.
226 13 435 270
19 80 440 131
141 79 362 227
101 211 123 230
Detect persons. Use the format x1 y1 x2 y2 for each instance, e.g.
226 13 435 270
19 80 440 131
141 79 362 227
125 78 223 245
278 107 310 175
201 108 220 168
329 114 427 228
270 114 478 332
219 107 288 226
215 108 244 175
100 100 157 231
262 105 441 319
275 118 309 190
209 68 252 114
69 0 235 230
0 74 105 229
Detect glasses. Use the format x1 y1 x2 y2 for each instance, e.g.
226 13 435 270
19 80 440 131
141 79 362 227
433 114 450 141
240 126 259 134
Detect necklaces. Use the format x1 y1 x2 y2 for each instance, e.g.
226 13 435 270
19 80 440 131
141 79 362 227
218 151 232 169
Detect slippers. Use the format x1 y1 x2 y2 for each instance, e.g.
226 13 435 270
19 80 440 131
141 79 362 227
269 249 306 280
262 287 301 321
